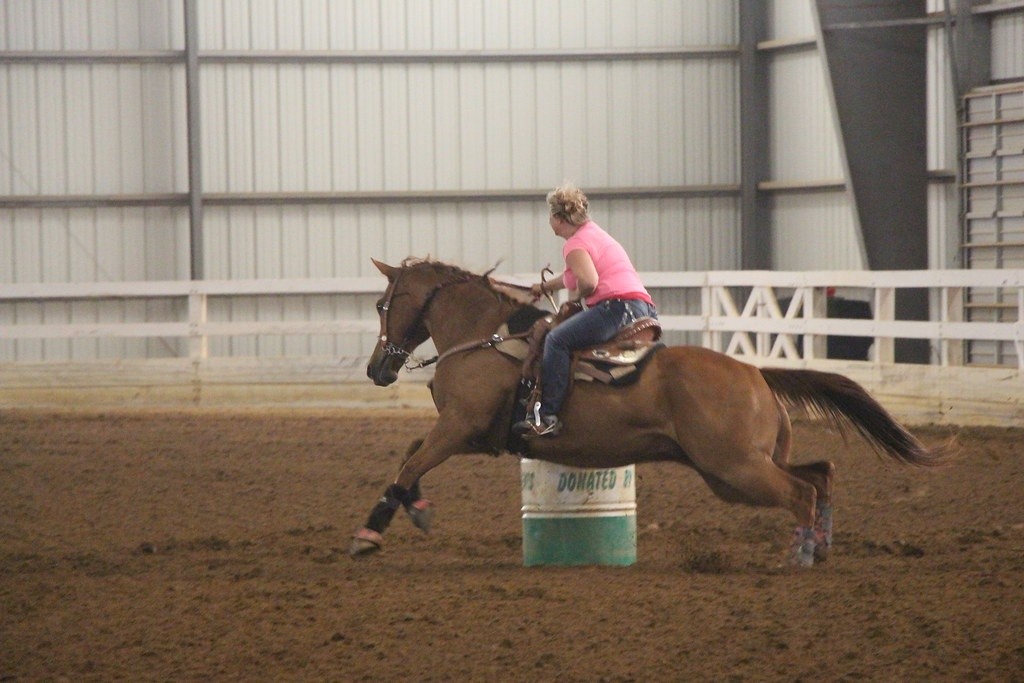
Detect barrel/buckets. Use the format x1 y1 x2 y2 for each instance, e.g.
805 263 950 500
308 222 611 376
520 457 637 566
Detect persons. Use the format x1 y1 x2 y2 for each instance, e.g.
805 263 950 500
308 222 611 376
510 184 659 440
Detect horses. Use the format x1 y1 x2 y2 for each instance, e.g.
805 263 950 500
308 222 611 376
347 254 970 570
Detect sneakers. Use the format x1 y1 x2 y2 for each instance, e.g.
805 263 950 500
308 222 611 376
510 413 563 440
